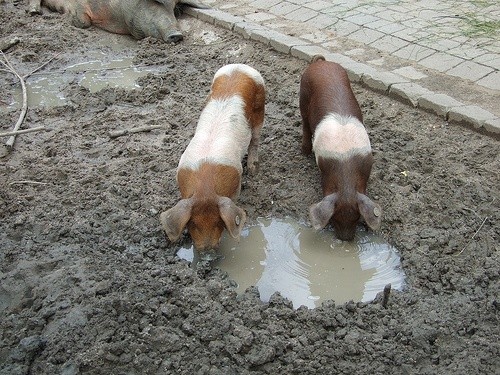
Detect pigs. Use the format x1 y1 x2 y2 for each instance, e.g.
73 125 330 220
28 0 212 44
299 54 380 243
161 64 267 263
